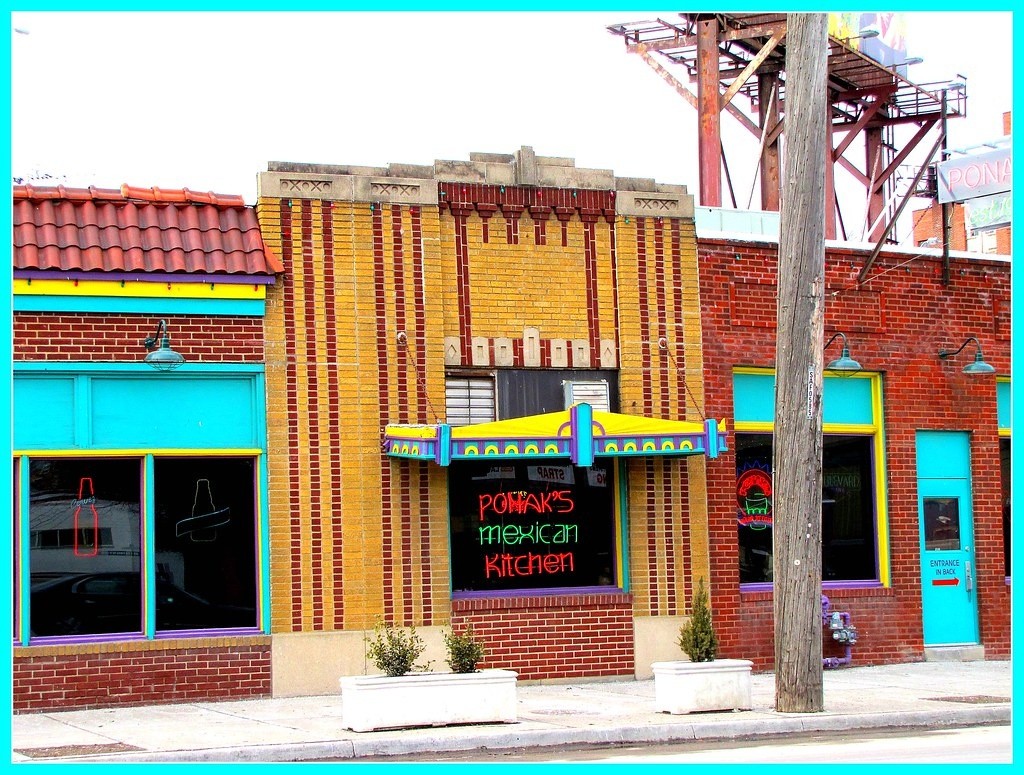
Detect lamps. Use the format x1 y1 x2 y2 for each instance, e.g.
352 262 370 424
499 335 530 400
144 320 186 373
937 337 997 381
885 55 924 69
824 332 864 377
917 79 966 91
839 29 881 41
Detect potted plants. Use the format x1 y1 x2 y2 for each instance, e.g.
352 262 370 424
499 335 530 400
649 576 753 716
338 614 520 733
